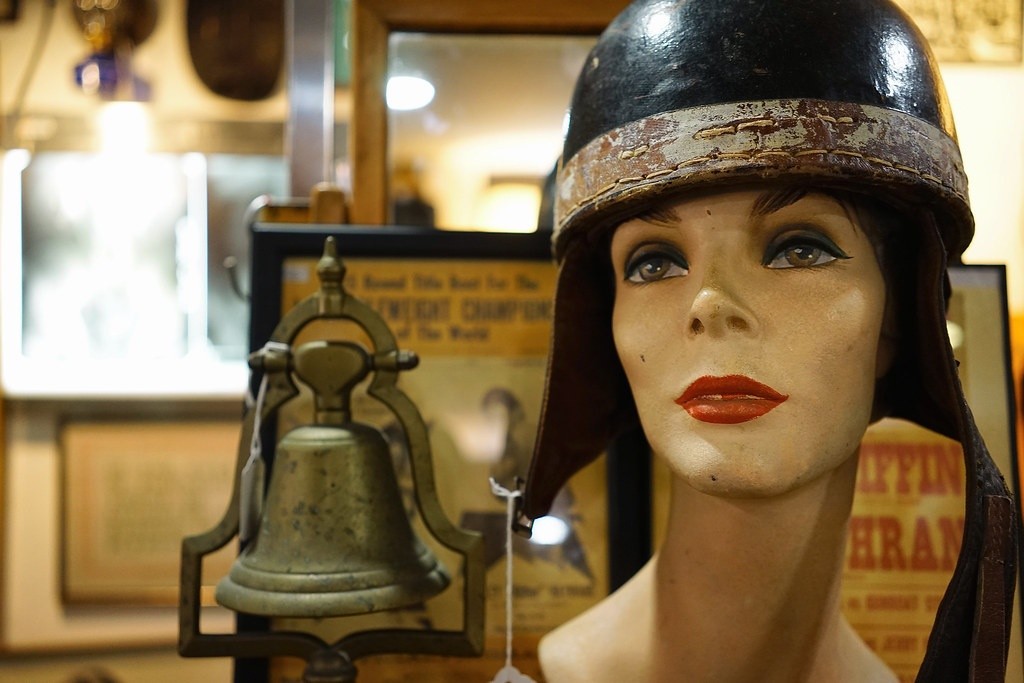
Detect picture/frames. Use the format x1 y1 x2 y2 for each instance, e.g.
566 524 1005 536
232 224 655 683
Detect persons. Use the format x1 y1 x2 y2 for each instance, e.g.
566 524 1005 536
517 2 1017 683
